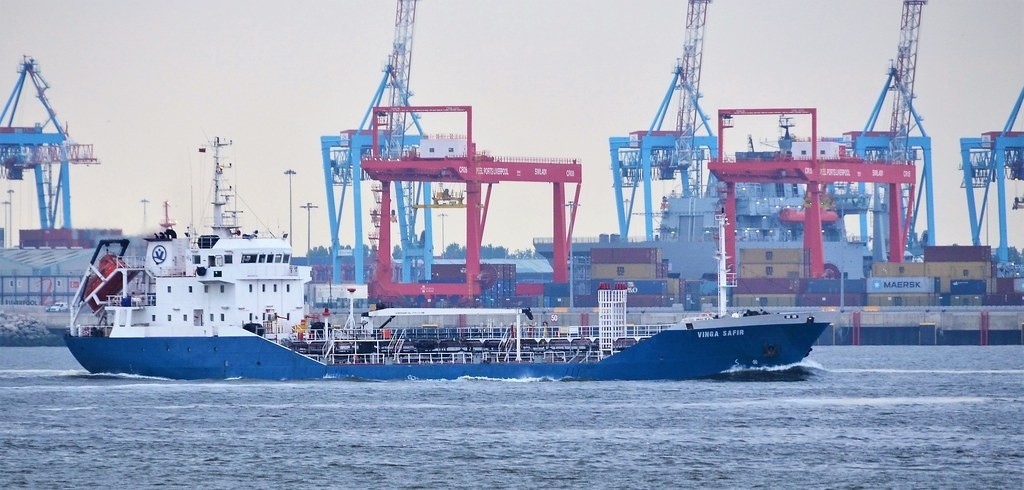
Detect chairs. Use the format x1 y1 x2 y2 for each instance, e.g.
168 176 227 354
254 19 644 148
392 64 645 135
416 338 638 362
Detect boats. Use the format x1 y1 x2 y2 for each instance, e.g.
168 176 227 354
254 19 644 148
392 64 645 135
65 232 834 383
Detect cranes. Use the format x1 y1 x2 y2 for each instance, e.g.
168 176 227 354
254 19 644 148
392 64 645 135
608 0 717 241
322 0 433 284
855 0 936 263
